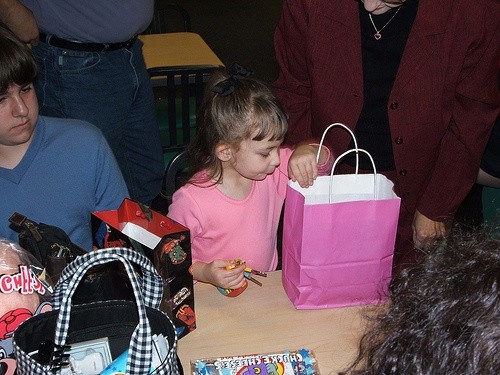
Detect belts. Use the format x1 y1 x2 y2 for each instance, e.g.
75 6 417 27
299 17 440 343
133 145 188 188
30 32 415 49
38 28 140 52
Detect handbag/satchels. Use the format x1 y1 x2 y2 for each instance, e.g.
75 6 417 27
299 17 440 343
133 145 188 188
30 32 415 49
90 197 196 341
283 122 402 311
8 212 89 285
12 246 187 375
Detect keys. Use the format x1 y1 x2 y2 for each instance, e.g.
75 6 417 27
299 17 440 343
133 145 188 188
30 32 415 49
243 268 268 287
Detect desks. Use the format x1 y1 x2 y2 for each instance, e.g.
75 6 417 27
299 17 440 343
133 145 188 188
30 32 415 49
179 267 398 375
139 31 225 86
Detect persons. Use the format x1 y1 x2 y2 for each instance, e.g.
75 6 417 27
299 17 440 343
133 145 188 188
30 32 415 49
0 0 165 213
0 35 131 255
276 0 500 271
165 66 334 289
338 218 500 375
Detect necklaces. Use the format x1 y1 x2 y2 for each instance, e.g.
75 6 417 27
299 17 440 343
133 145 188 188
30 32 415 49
369 7 402 41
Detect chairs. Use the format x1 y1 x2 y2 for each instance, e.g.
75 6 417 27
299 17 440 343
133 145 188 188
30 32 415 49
143 4 190 33
147 62 224 213
